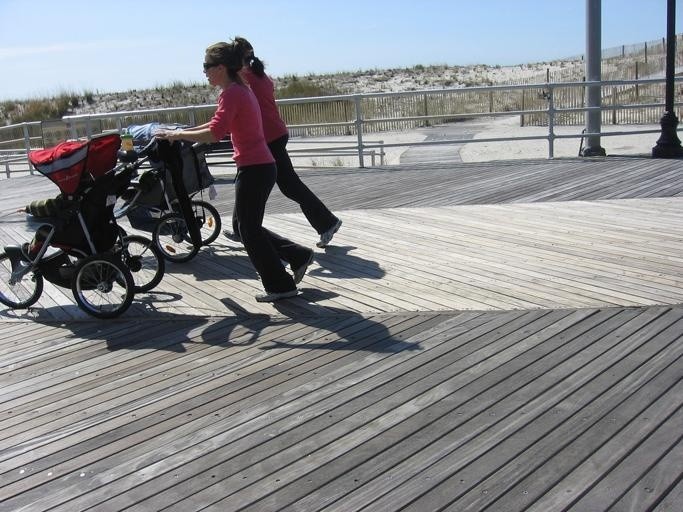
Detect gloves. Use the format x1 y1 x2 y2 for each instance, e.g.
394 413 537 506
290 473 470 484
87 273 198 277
154 128 182 142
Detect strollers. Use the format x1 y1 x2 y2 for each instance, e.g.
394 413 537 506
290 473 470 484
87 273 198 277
0 136 164 316
111 130 222 263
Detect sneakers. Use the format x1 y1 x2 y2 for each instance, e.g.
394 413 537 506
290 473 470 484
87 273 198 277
293 249 313 284
255 289 298 302
223 229 241 242
316 219 342 248
21 242 34 262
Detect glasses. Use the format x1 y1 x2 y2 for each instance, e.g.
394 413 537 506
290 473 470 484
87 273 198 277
203 63 218 70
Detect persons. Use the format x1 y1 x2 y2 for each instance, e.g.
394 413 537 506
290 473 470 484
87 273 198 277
221 36 343 250
15 191 81 267
153 43 316 303
